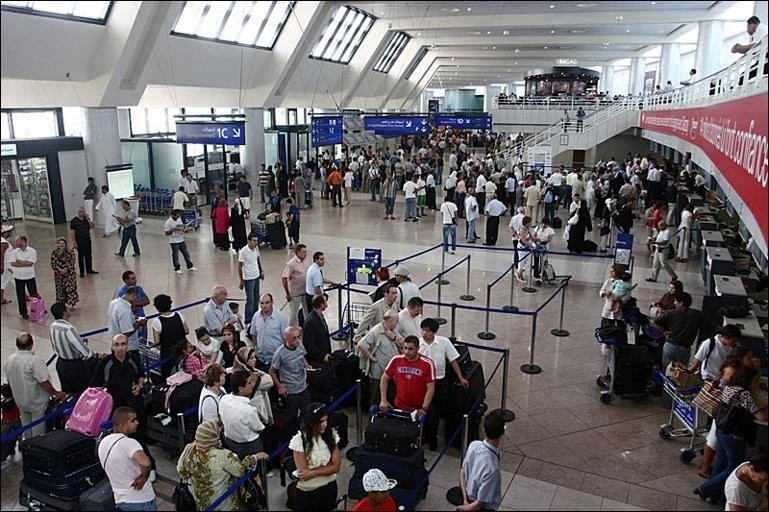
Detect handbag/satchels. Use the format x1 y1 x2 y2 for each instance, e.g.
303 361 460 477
553 212 625 251
30 293 44 322
666 361 752 439
452 217 458 225
251 209 287 248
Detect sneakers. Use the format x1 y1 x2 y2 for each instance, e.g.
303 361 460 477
645 277 657 282
384 213 427 222
671 276 678 280
187 267 197 271
87 271 98 274
114 252 123 257
176 269 183 275
80 274 85 279
132 253 140 256
465 236 490 245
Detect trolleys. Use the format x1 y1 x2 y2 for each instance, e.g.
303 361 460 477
133 338 165 381
332 301 372 347
61 408 114 444
183 207 200 234
532 242 572 287
597 321 655 405
133 184 176 216
658 368 710 464
250 217 271 248
348 403 429 511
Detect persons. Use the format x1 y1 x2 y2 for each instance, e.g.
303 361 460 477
163 125 704 284
561 108 570 134
731 16 769 85
70 206 99 279
746 25 755 43
0 224 31 307
95 185 122 238
598 263 768 511
82 177 98 222
50 237 80 311
113 199 141 257
498 70 697 107
9 234 48 320
1 285 506 512
574 107 585 133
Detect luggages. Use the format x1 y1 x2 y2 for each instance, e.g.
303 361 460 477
261 343 486 507
0 372 204 511
539 252 556 282
593 297 664 395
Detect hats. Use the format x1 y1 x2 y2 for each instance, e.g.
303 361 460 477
392 265 410 276
